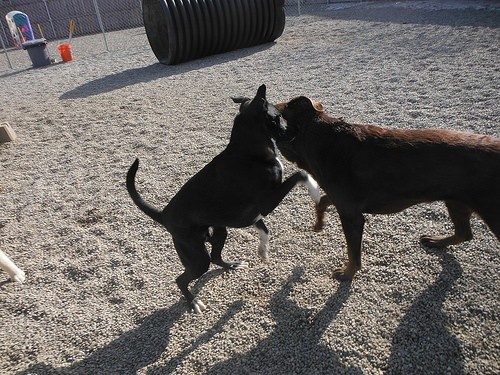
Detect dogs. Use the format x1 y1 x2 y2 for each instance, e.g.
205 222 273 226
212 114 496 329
271 94 500 283
126 83 321 315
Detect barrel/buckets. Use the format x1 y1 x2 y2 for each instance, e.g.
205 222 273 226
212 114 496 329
57 43 73 62
